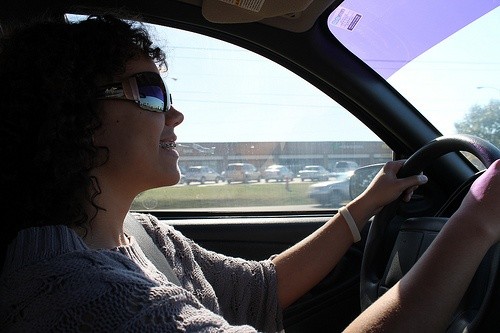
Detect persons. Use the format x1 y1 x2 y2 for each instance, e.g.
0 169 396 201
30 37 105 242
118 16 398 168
0 13 500 333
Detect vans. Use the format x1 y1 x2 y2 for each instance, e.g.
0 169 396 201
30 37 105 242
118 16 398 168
225 163 261 184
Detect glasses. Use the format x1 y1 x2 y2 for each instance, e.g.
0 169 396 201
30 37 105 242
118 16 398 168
97 69 173 113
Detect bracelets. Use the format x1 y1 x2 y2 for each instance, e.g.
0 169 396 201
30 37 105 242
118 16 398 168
339 208 362 242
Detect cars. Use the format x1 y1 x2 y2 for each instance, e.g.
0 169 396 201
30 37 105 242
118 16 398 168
265 164 294 183
300 165 329 182
309 172 356 207
185 166 221 185
178 174 185 184
335 161 358 177
